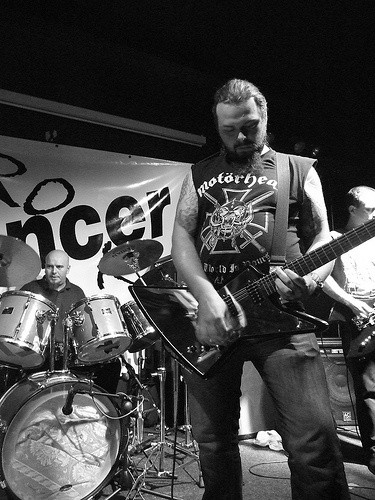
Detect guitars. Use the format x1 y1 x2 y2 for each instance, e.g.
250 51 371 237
129 215 375 379
328 299 375 359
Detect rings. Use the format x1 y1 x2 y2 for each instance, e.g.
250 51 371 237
288 291 293 297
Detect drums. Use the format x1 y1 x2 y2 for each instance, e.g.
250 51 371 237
0 291 59 370
63 295 132 363
0 372 123 500
121 300 161 353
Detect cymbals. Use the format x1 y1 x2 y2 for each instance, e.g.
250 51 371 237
98 240 163 275
0 235 41 287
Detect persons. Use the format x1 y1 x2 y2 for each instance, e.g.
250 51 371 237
272 128 308 157
321 186 375 477
20 250 87 343
172 78 352 500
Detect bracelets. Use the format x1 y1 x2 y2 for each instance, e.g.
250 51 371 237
309 272 324 299
